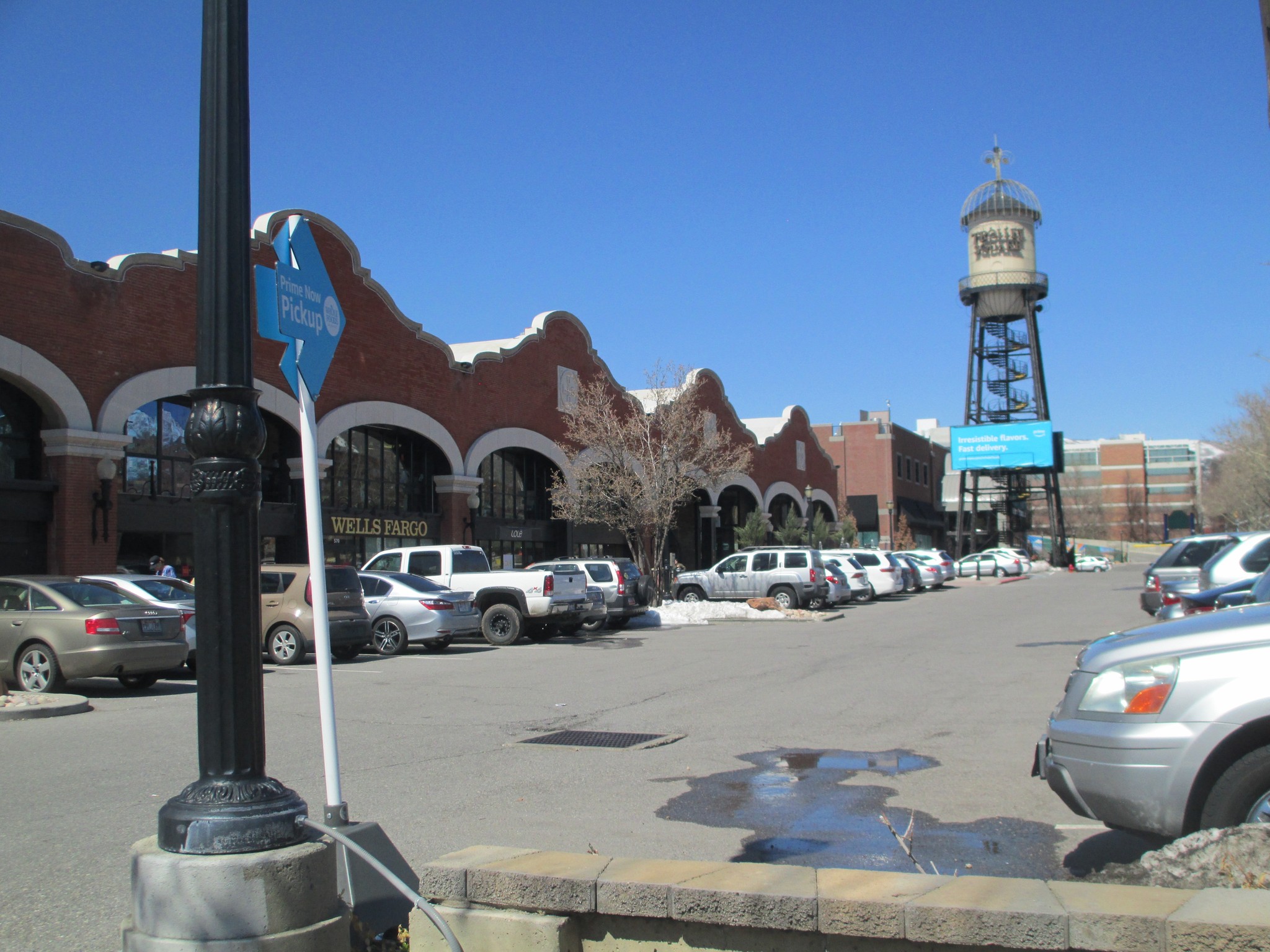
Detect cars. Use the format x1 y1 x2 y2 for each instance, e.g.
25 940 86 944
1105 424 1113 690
894 555 922 591
821 554 871 600
1136 531 1270 622
26 573 195 675
0 573 188 694
983 547 1031 574
907 556 944 590
891 548 956 582
1074 556 1109 573
1029 603 1270 832
823 562 852 606
953 553 1022 578
355 570 484 656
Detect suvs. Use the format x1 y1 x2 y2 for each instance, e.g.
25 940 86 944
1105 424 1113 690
670 545 831 610
819 548 904 598
185 557 370 665
523 556 657 637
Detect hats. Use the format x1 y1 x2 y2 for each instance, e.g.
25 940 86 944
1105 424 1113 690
150 556 160 569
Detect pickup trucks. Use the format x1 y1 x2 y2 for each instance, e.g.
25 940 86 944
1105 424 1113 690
357 545 589 646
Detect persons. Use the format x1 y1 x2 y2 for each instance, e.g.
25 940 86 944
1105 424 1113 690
672 558 686 580
170 555 183 579
149 555 177 597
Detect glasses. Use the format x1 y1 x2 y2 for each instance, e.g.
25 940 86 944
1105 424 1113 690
160 560 165 564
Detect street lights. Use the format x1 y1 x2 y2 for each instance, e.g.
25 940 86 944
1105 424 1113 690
804 485 814 547
886 500 895 550
